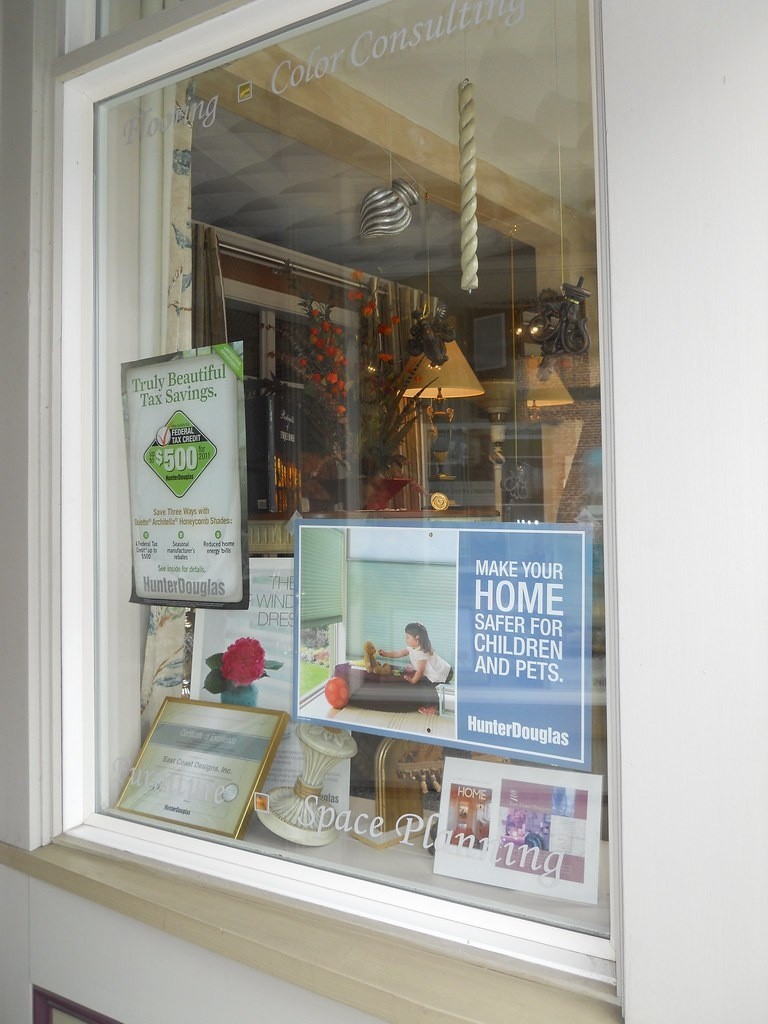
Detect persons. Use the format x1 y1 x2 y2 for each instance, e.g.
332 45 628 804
378 623 453 716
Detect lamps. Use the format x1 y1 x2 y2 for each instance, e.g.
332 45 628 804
396 340 484 481
523 356 574 424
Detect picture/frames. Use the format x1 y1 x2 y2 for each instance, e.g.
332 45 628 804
113 696 292 840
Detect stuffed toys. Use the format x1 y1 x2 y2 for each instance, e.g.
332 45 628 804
363 640 392 675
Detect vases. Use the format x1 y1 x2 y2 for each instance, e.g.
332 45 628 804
221 683 259 707
317 479 409 511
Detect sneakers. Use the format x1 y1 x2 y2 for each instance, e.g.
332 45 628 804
417 705 439 717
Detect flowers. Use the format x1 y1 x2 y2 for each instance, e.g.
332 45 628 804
203 636 284 695
251 259 440 480
